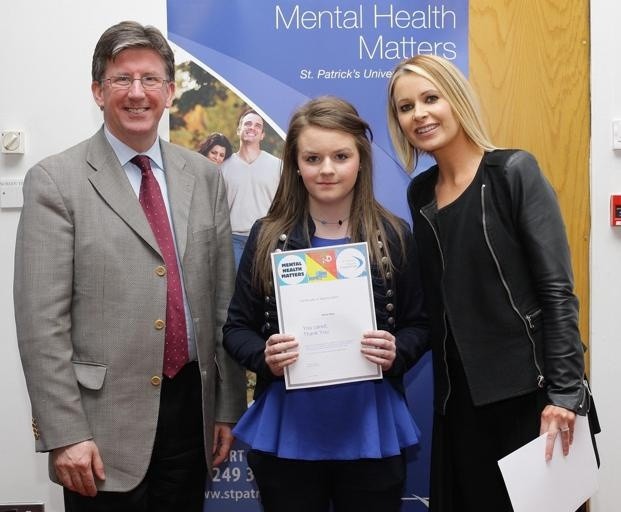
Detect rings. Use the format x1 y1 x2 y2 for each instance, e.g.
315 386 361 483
561 426 569 432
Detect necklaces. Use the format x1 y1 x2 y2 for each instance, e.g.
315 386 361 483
310 215 349 225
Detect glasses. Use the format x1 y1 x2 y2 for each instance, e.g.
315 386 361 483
100 75 170 90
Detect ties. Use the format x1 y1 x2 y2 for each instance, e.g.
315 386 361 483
129 155 189 379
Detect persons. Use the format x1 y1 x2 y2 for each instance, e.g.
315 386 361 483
387 54 599 512
220 110 285 273
222 95 429 512
13 21 247 512
197 132 233 164
